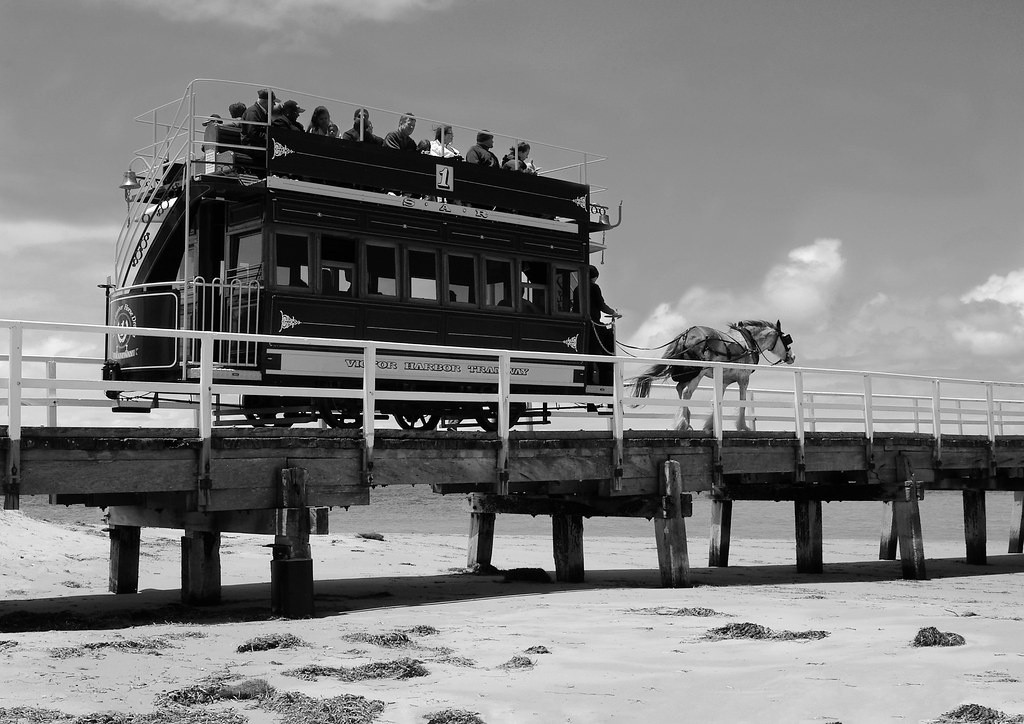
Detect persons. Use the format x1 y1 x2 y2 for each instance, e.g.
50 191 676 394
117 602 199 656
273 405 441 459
572 265 622 385
199 87 557 222
344 263 533 311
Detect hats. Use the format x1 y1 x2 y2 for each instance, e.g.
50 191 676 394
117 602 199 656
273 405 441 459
477 129 494 143
590 265 599 278
257 88 281 103
202 114 223 126
283 99 305 113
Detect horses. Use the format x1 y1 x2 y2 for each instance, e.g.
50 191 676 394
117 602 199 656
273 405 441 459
629 319 796 435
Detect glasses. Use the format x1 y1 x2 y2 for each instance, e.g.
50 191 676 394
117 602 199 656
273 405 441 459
446 132 453 135
358 114 366 118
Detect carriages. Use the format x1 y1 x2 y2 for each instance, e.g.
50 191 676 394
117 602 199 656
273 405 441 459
98 75 798 430
